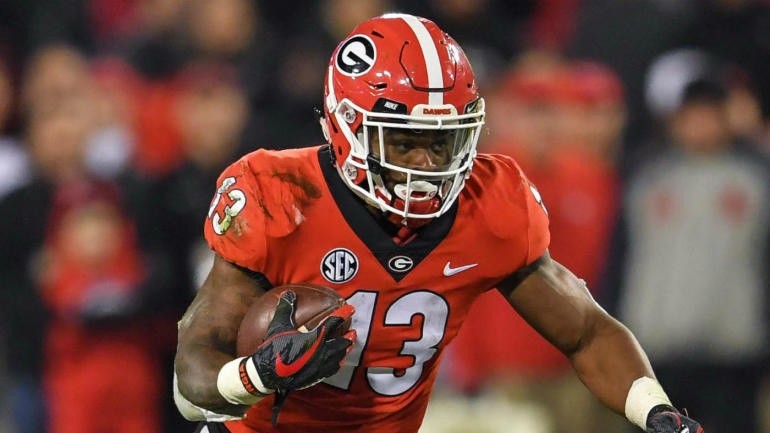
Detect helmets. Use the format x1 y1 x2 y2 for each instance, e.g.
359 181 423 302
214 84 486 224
324 12 486 217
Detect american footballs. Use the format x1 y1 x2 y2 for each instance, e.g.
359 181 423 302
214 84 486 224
235 282 352 358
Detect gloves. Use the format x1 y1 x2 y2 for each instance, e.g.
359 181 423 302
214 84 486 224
252 289 357 390
645 405 704 433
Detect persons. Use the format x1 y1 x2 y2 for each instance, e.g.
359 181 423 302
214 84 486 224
0 0 770 433
176 13 704 433
